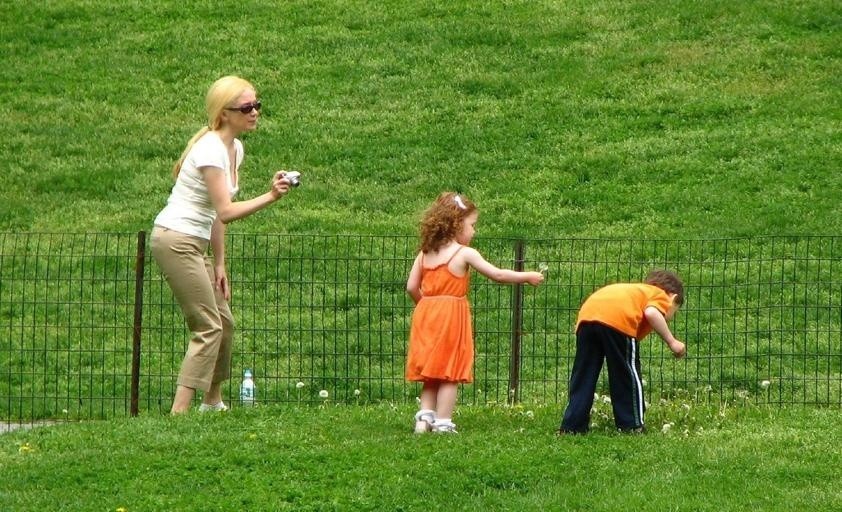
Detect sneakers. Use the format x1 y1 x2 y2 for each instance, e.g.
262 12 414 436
196 402 229 412
414 420 458 434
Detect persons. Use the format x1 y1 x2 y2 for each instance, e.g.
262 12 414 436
150 75 291 418
407 191 544 435
561 269 687 434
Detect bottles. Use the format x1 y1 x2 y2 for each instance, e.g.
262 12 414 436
241 370 256 404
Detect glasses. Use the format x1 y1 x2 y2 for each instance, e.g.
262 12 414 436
224 103 261 114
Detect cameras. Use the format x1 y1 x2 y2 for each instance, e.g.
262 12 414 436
283 171 301 187
